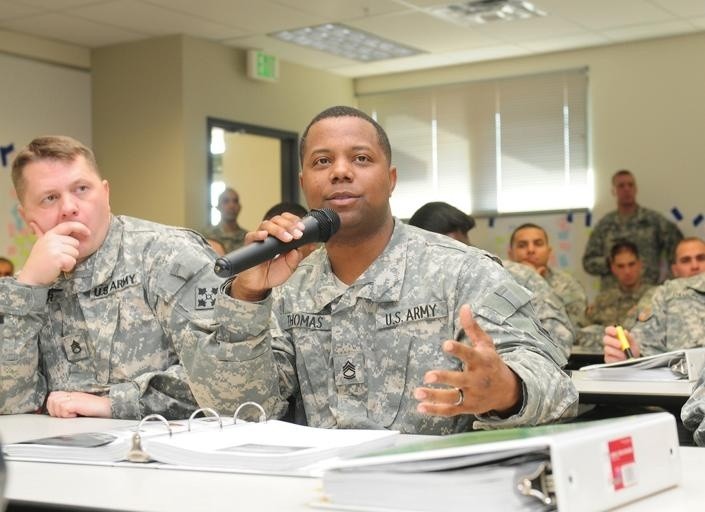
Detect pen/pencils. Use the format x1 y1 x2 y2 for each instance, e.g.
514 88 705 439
615 323 636 360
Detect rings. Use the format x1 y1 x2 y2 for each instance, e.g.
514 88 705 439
455 389 466 409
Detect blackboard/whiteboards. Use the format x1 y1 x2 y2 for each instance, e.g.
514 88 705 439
401 208 601 307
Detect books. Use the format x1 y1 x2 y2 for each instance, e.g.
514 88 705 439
581 343 705 383
1 393 406 478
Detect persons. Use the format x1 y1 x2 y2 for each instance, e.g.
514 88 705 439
0 256 13 280
582 168 684 292
588 240 658 323
261 201 314 263
0 133 231 418
200 185 250 255
603 269 705 364
508 221 588 332
190 105 579 438
667 236 704 277
409 200 575 366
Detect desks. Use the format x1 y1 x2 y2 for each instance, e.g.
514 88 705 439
0 414 705 512
571 363 704 412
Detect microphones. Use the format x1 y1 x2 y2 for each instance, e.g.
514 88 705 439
213 207 340 278
